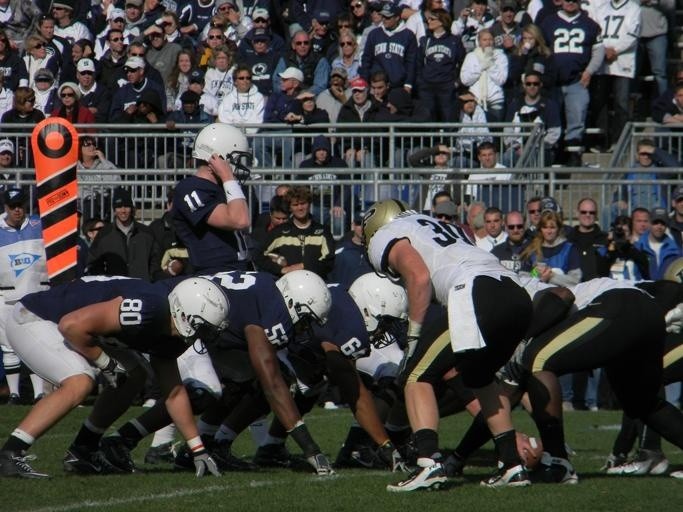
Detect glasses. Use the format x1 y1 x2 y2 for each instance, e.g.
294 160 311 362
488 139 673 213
340 41 352 46
254 18 267 23
210 23 223 28
109 37 125 41
80 71 92 75
280 77 294 82
350 3 362 9
209 34 222 40
525 81 539 87
51 6 63 11
381 15 396 20
131 52 145 57
529 209 542 214
566 0 577 4
465 99 475 103
337 24 350 29
427 15 440 21
89 226 104 231
31 41 48 48
219 6 232 11
254 39 267 44
296 40 309 45
507 224 524 229
60 92 74 98
124 68 137 72
236 76 251 81
26 96 36 104
580 210 597 215
8 202 23 208
1 38 6 43
435 213 452 220
38 79 48 83
82 141 95 147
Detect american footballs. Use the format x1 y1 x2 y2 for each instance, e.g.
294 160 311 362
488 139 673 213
494 431 543 476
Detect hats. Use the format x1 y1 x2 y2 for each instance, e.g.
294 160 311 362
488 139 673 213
378 3 402 18
540 196 561 213
109 8 126 21
650 207 670 226
52 0 74 10
435 200 458 216
310 8 332 24
33 68 55 81
278 66 305 83
252 8 269 20
3 188 28 205
180 90 201 103
187 67 204 82
122 57 146 69
672 188 683 200
500 1 518 14
77 58 96 72
330 66 348 80
125 0 142 7
58 82 82 100
0 139 15 155
351 78 368 91
112 187 133 208
251 27 272 41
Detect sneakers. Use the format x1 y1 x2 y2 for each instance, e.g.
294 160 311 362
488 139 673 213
531 451 578 484
63 441 126 475
651 451 669 475
6 393 21 405
479 461 530 487
253 445 306 470
607 449 669 475
172 445 262 474
175 433 214 469
331 444 386 469
0 450 49 479
202 435 260 473
98 435 144 475
370 438 418 467
386 457 448 492
668 470 682 478
408 449 463 478
145 443 179 466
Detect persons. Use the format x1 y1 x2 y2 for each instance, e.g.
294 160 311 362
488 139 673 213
253 66 305 168
298 136 350 224
336 15 353 41
88 191 163 408
627 208 652 249
270 29 331 105
615 139 681 220
534 2 604 160
630 216 682 276
100 29 130 74
173 124 252 269
243 28 278 69
109 56 162 164
31 14 70 71
81 218 105 270
363 3 418 97
329 32 362 80
570 280 680 478
643 0 677 116
295 90 331 147
167 50 192 98
261 188 335 272
70 39 103 83
27 69 58 114
370 73 392 102
660 185 683 247
428 193 454 232
466 142 513 199
338 81 394 174
656 80 682 123
510 69 563 164
487 0 526 53
76 138 122 215
466 198 488 227
121 2 144 26
148 189 193 284
335 214 382 277
511 26 549 97
431 276 683 486
454 92 499 157
177 71 206 104
478 208 508 252
2 89 46 167
73 59 103 118
204 28 236 61
459 29 507 145
272 185 290 207
0 139 16 187
450 1 496 38
207 0 245 29
134 24 183 77
218 69 266 137
407 146 463 211
175 273 414 477
317 64 351 148
49 0 91 48
118 42 160 87
308 10 332 65
0 29 28 92
395 5 426 47
0 0 45 39
155 12 195 47
20 35 59 86
101 269 335 476
245 9 274 41
261 196 290 240
570 199 609 264
525 198 547 243
492 211 534 275
383 89 432 141
201 44 234 120
4 275 229 479
415 9 466 148
431 202 475 249
361 200 531 493
48 83 94 156
0 189 46 404
95 9 140 59
604 215 648 275
529 210 582 280
586 0 642 135
406 2 443 44
157 92 215 174
350 1 374 34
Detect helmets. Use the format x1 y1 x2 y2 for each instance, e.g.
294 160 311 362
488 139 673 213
192 122 253 185
167 277 229 353
350 271 412 353
277 268 332 340
360 198 411 247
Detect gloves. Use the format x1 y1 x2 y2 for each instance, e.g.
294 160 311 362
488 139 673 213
599 454 626 473
397 336 418 377
304 452 339 479
325 349 361 414
190 448 224 478
380 441 412 474
103 357 131 392
